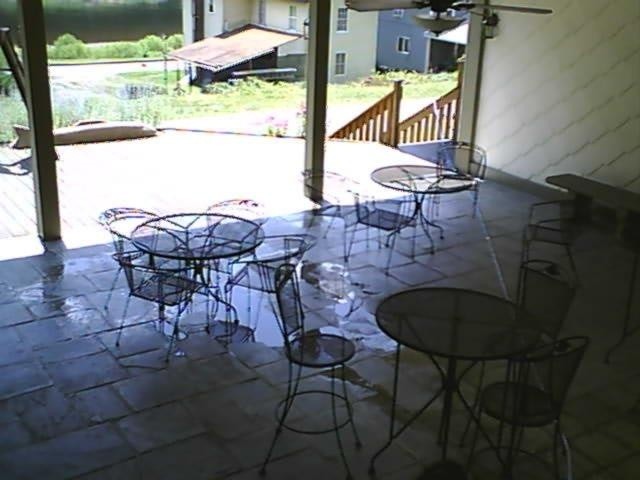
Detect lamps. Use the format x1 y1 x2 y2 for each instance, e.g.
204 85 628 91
414 2 465 39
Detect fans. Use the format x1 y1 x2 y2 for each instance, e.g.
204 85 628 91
343 2 557 19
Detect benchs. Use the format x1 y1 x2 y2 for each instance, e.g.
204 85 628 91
541 172 640 255
